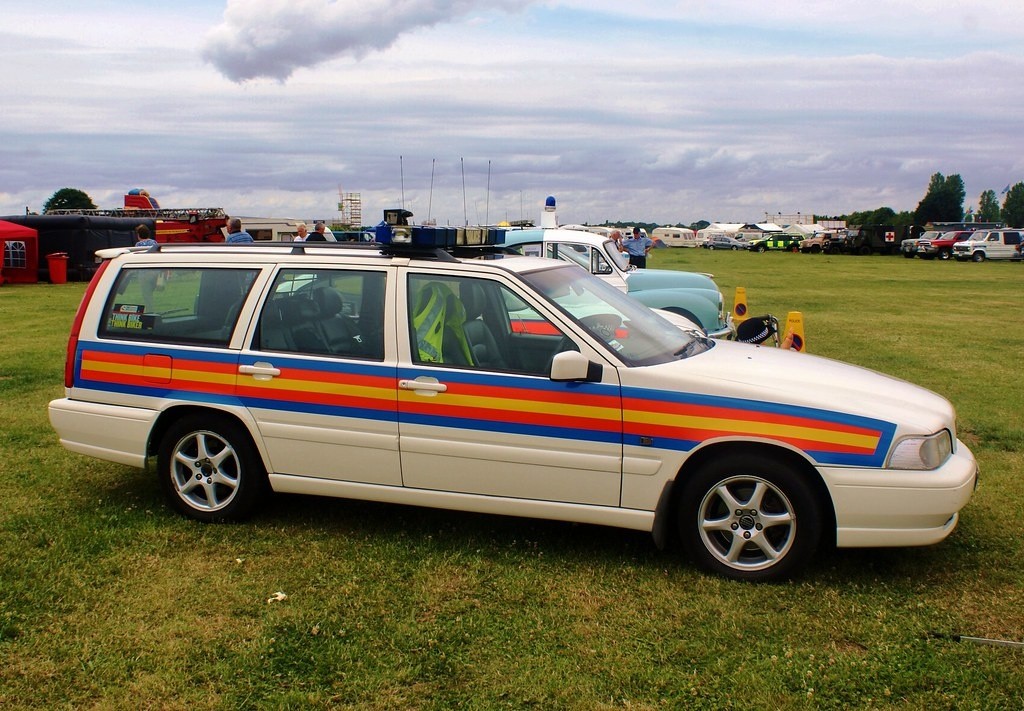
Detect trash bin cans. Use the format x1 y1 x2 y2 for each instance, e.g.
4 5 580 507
45 252 69 284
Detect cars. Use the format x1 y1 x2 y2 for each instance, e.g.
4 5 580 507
706 236 747 251
273 227 734 342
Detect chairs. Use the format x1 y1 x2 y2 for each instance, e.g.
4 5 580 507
415 280 508 369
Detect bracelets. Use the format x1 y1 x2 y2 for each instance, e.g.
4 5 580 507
648 248 650 250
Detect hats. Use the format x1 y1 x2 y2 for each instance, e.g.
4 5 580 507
734 317 774 345
633 227 640 233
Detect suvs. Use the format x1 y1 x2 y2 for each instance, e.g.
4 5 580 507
799 229 841 254
45 209 978 584
951 228 1024 263
900 231 946 259
915 231 973 261
702 233 725 250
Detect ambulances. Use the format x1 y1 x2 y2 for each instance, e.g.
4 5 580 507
747 233 805 253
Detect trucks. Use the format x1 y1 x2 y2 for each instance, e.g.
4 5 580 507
821 223 910 255
735 232 764 243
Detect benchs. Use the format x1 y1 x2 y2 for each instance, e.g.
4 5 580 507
222 287 384 360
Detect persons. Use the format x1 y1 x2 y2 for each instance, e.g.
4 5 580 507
611 230 623 252
136 225 159 311
813 230 816 237
226 218 254 243
294 224 310 242
621 227 656 268
306 223 326 242
1020 234 1024 256
